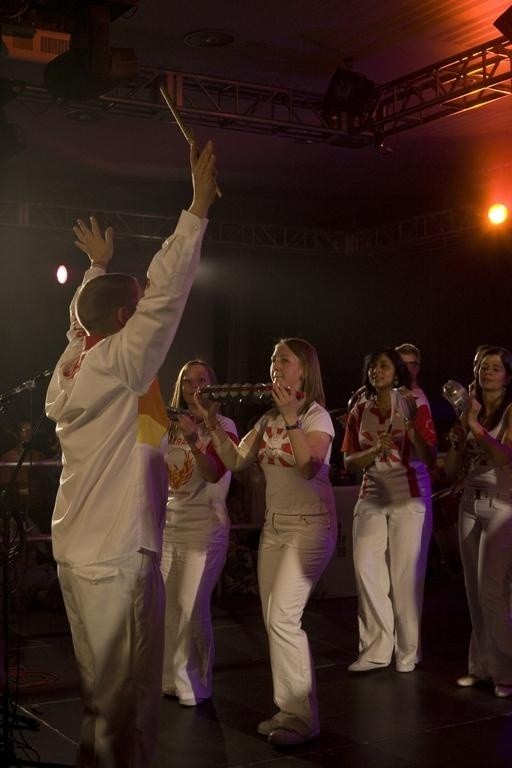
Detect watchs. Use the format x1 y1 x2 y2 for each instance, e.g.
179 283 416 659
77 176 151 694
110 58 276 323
286 419 303 431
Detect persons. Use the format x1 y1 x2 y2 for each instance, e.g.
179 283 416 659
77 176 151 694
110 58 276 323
0 419 46 482
44 138 218 765
472 345 491 380
342 347 437 676
159 360 237 708
445 349 512 697
195 336 339 746
394 344 432 417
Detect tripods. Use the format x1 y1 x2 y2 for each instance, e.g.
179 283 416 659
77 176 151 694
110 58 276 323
0 482 83 763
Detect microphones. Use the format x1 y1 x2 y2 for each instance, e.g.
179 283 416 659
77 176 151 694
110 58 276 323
42 368 55 377
4 377 38 403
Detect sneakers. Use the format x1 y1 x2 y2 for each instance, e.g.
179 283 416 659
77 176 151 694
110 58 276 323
347 659 415 672
457 675 512 697
259 719 318 744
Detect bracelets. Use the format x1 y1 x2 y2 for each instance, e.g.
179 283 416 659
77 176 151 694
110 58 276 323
192 444 205 456
206 421 221 433
